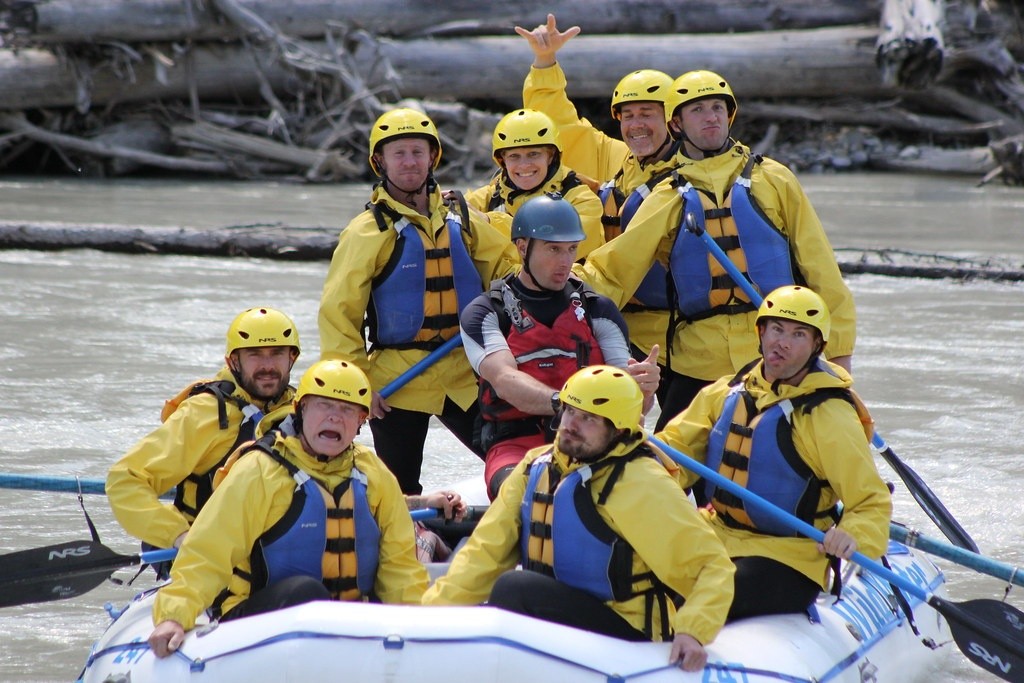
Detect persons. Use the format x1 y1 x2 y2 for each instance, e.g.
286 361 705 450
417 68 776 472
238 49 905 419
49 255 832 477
650 282 893 626
513 10 676 404
104 304 468 561
149 357 431 657
455 184 660 501
317 108 521 489
438 106 606 266
572 68 858 430
419 364 737 673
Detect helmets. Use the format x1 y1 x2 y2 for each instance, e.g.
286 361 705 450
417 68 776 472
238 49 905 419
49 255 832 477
224 306 300 359
664 69 738 140
753 284 830 357
511 193 587 242
559 364 644 440
291 359 372 421
369 108 442 178
611 70 673 122
492 108 562 170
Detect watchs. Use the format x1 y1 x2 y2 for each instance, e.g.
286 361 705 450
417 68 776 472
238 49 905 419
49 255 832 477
551 391 560 413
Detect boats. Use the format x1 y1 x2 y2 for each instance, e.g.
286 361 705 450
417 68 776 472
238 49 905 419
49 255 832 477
81 524 965 680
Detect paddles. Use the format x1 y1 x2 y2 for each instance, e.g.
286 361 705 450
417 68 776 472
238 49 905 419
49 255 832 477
686 212 983 573
378 332 462 400
833 503 1024 587
637 423 1024 683
0 495 456 607
1 473 489 518
